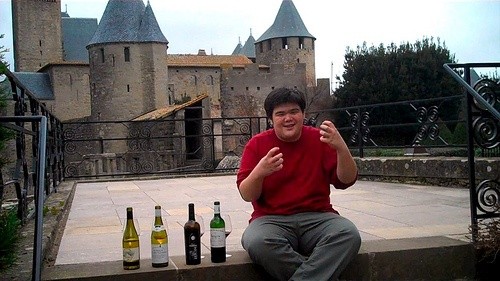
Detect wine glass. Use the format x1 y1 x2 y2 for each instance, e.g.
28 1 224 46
194 215 205 259
221 215 231 257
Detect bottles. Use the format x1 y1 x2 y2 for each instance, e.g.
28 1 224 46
122 207 140 270
151 206 168 268
210 202 226 263
184 203 201 265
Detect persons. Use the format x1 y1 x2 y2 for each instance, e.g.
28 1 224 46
236 87 362 280
